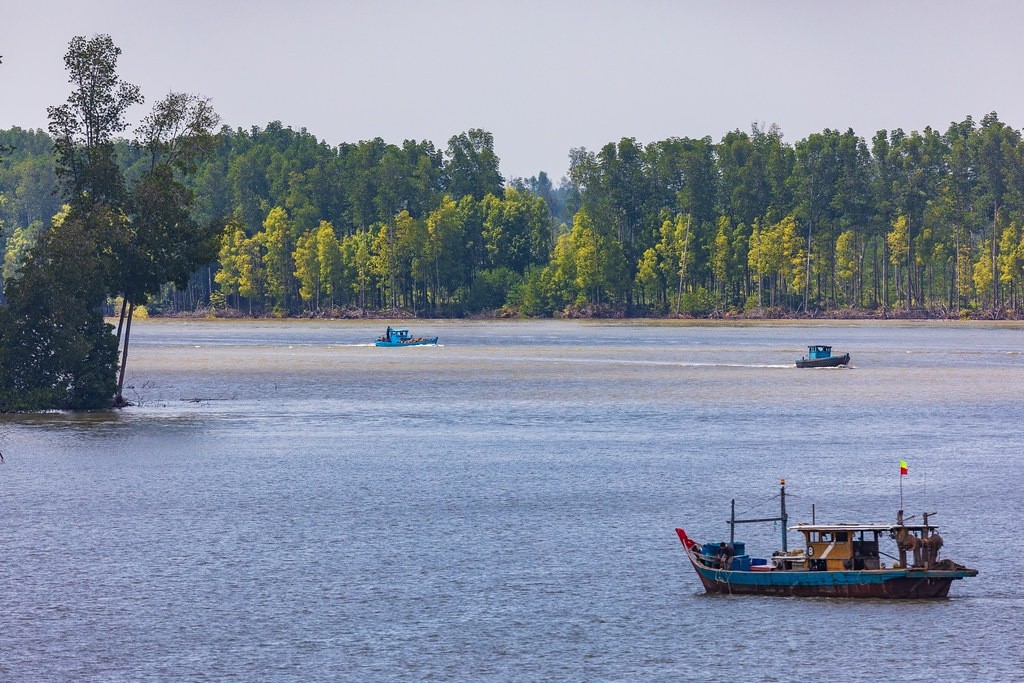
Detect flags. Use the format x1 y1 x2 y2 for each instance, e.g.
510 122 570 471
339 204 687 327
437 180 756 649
900 459 908 475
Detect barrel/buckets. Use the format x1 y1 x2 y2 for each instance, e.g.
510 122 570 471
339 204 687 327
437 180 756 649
701 541 751 572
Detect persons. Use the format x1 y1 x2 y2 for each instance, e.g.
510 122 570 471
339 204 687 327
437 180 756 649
386 326 392 339
719 542 733 570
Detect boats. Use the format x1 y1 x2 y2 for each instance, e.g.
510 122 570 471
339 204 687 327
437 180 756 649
374 329 438 347
675 460 978 597
795 345 850 368
